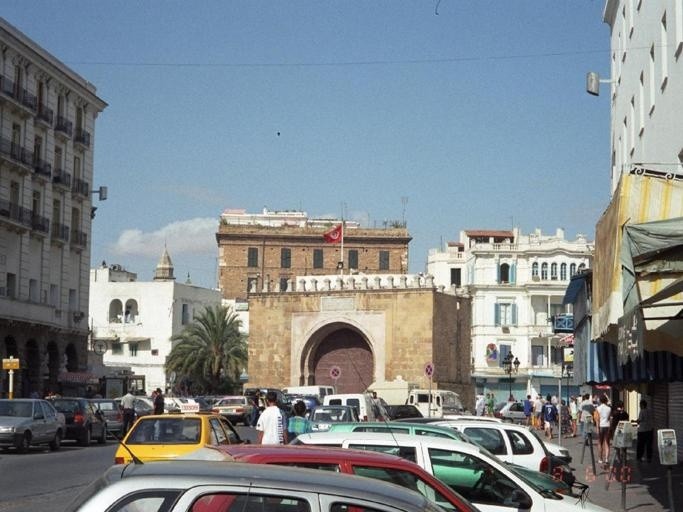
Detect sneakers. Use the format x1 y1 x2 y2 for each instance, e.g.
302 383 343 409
534 426 577 443
640 456 646 461
647 458 652 463
597 460 603 464
605 459 609 463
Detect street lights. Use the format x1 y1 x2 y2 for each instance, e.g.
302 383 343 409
502 351 519 401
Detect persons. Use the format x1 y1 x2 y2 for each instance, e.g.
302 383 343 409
152 390 157 403
119 388 135 438
28 387 39 398
84 382 105 398
257 391 265 415
152 387 163 414
286 401 312 443
255 391 288 446
249 388 260 428
483 392 653 465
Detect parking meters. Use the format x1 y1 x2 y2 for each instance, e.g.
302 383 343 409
657 429 678 511
612 421 632 511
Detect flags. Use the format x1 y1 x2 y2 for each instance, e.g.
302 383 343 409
322 224 341 243
